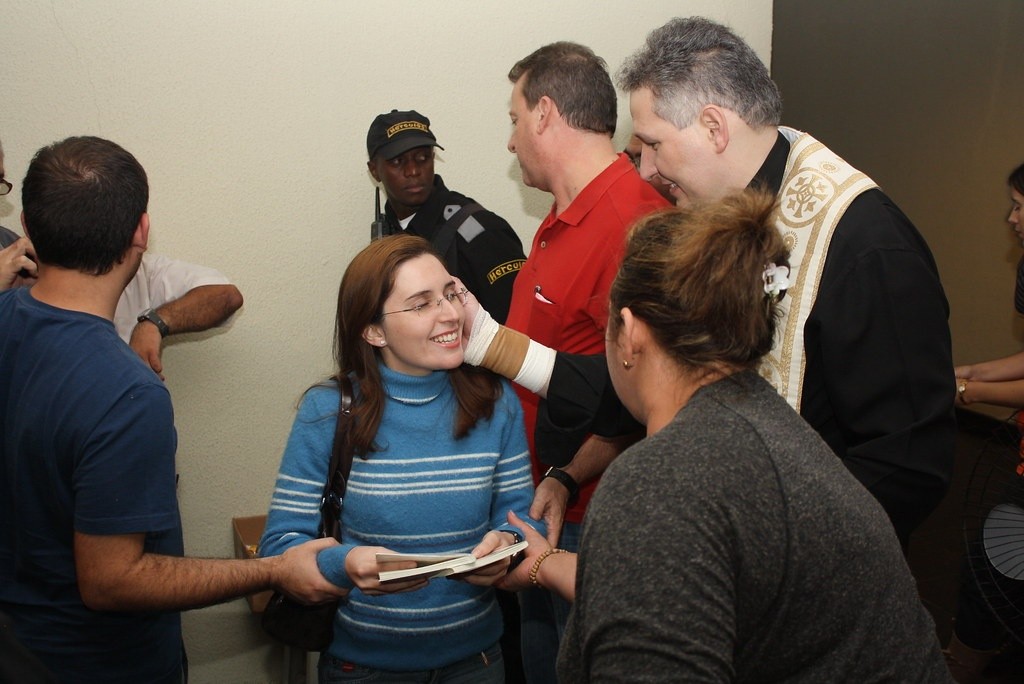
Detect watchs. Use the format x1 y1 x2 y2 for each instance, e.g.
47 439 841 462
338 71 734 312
137 308 170 339
958 382 969 405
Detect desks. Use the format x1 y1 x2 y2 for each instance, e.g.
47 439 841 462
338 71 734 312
233 515 306 684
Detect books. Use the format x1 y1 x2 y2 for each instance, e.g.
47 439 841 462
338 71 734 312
375 540 529 582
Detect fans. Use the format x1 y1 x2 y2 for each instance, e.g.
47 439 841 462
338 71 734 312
960 407 1024 646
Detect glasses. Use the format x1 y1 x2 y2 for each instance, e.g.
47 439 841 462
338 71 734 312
382 287 470 319
0 177 12 195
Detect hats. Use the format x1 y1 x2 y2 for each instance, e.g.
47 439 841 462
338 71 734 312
366 109 445 161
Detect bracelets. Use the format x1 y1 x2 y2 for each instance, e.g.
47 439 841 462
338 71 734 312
510 532 521 562
540 466 581 504
529 548 568 588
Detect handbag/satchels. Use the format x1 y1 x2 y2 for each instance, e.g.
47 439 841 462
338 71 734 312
257 374 357 651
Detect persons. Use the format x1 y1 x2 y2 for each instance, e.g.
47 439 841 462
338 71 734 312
452 17 956 542
950 163 1024 408
0 141 38 294
0 136 350 684
365 109 527 324
496 188 953 684
256 233 549 684
502 42 676 684
113 255 244 381
